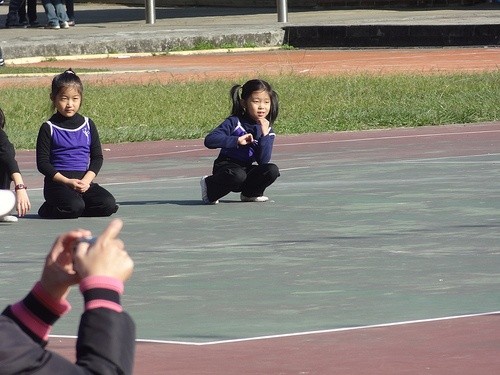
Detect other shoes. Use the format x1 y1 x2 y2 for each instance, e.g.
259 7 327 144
0 58 4 66
31 20 41 27
60 21 69 28
45 23 60 29
61 17 75 26
21 21 31 28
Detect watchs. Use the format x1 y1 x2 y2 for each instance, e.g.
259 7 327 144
14 184 27 191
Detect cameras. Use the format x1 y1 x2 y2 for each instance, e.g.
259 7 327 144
71 235 96 272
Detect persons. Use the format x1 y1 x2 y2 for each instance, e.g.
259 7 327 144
0 0 78 30
0 110 31 222
0 47 5 66
199 78 280 205
0 216 138 375
35 69 119 218
